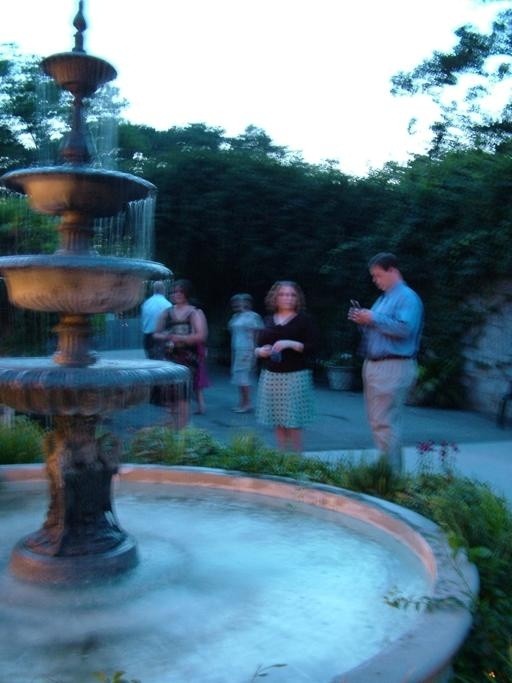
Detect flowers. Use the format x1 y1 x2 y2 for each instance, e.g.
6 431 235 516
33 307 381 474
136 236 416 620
317 347 361 369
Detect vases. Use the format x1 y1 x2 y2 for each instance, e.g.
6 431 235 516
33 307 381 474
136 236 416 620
325 363 354 391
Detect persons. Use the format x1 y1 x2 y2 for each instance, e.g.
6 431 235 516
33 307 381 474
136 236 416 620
251 280 322 458
224 293 259 413
139 277 210 429
346 252 424 479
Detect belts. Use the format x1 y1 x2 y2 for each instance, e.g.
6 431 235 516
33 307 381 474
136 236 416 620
367 354 416 361
